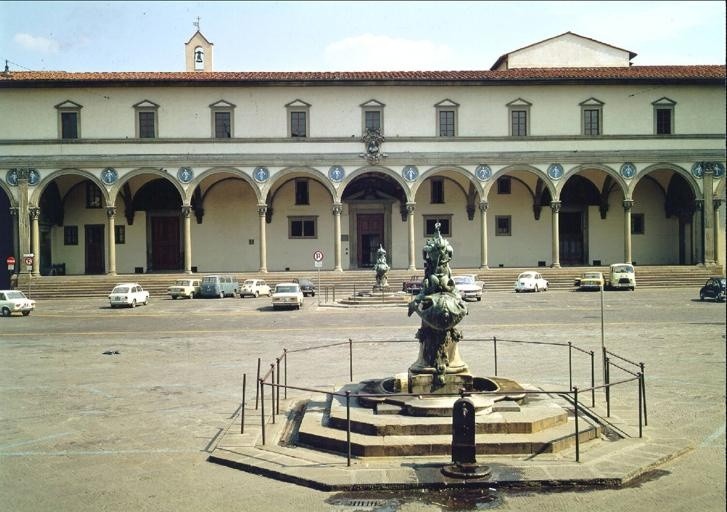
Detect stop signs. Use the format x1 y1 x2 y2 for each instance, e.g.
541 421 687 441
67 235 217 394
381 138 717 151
7 257 15 265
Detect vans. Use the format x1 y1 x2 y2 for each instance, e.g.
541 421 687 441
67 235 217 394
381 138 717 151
608 263 636 291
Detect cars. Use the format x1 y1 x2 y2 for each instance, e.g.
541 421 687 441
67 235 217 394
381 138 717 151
699 276 726 303
402 275 425 294
452 274 486 301
107 282 150 308
514 271 549 292
575 271 606 290
166 275 315 310
0 290 36 317
